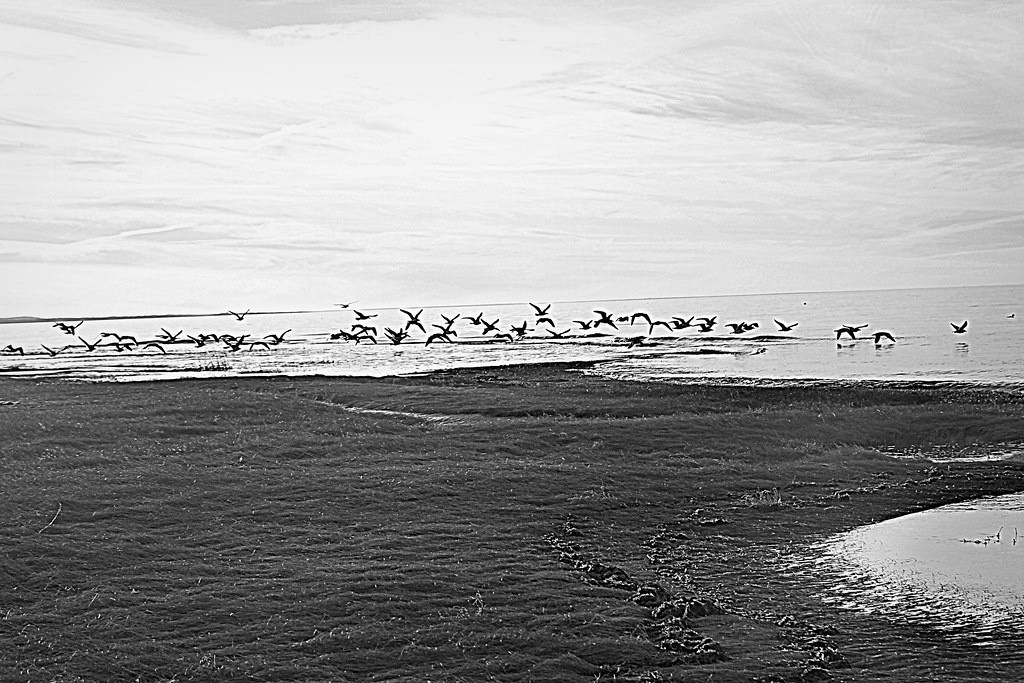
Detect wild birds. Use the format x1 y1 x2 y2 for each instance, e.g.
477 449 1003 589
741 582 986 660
0 301 1015 359
